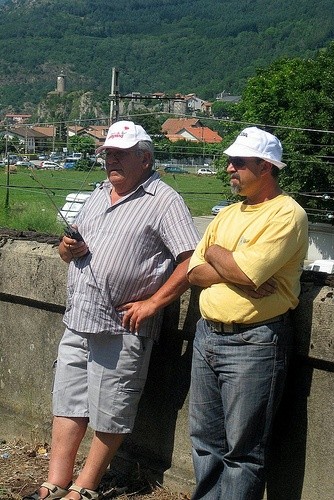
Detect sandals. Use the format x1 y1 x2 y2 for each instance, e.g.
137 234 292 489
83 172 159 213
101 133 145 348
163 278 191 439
22 479 73 500
61 483 104 500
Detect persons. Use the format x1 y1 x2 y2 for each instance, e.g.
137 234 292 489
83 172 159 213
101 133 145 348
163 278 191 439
20 120 202 500
187 126 309 500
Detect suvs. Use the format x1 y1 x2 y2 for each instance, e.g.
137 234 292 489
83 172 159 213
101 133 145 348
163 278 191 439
163 167 188 175
198 167 217 175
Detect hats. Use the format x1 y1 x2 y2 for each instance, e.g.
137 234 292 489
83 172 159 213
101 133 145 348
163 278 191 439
95 121 153 154
223 127 287 170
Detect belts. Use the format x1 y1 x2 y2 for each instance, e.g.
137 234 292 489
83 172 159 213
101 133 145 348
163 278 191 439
205 311 289 333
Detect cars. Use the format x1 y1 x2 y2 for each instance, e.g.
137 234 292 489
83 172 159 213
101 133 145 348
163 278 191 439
0 151 102 170
211 200 236 215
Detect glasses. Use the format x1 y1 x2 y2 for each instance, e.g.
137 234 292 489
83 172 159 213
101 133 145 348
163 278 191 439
226 156 263 168
101 149 143 160
